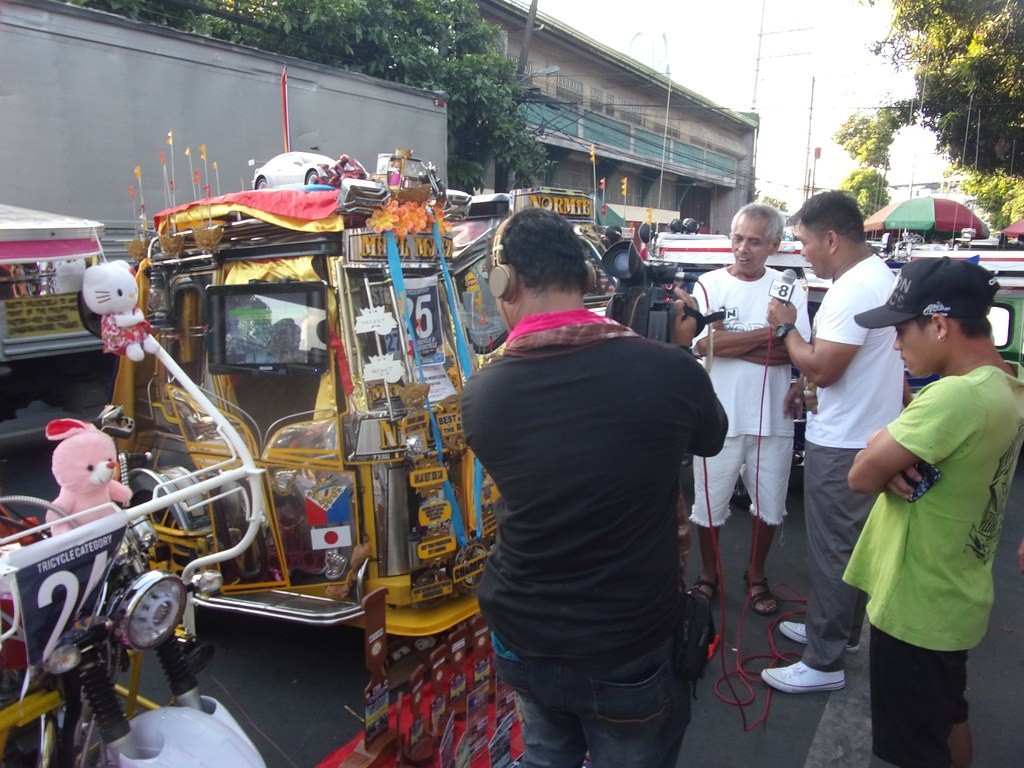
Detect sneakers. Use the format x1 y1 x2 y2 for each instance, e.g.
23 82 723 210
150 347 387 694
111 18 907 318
761 661 845 693
780 621 859 653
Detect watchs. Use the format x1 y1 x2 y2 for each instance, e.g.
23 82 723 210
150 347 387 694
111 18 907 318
774 321 795 339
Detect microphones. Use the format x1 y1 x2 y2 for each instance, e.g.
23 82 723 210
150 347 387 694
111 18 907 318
768 269 797 304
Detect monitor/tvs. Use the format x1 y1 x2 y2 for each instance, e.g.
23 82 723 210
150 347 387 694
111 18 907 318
205 282 329 375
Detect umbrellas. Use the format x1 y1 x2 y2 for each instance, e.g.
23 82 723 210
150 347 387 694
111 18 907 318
862 194 992 241
1003 218 1024 238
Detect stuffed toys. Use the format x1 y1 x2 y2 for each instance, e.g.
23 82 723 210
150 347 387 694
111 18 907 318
40 415 130 541
51 257 84 295
81 260 166 364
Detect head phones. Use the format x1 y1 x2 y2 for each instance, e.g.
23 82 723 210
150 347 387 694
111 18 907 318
489 215 597 301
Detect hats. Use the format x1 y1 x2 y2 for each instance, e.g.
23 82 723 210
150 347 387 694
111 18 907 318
854 256 1001 329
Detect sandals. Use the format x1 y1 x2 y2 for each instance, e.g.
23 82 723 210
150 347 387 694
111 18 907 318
687 575 720 606
743 569 779 615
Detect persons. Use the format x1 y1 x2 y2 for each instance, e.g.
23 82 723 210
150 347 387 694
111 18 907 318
461 208 731 768
688 204 815 618
761 192 908 693
844 259 1023 766
668 215 700 235
220 294 304 368
